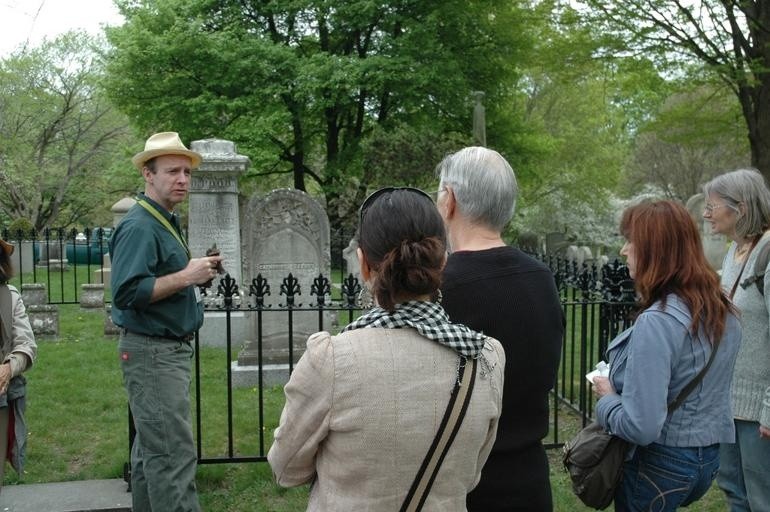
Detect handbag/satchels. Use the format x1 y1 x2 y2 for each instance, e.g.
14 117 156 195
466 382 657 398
561 421 630 511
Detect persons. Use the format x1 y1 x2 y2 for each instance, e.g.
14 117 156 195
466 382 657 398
434 144 568 510
0 236 38 512
266 187 507 512
109 131 223 512
591 197 744 512
700 165 770 512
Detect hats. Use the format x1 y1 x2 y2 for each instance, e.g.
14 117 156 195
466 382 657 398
133 131 202 167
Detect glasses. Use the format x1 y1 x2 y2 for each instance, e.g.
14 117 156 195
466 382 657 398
706 201 734 211
357 186 434 242
